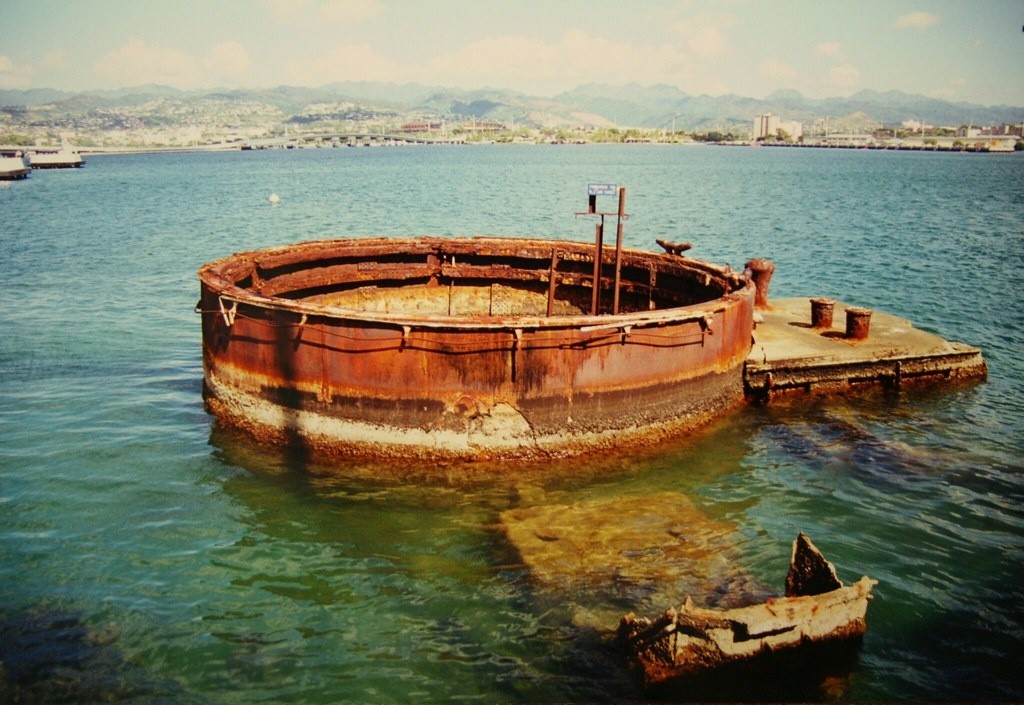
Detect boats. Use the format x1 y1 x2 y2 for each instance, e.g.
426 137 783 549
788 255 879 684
28 136 89 168
0 146 32 181
